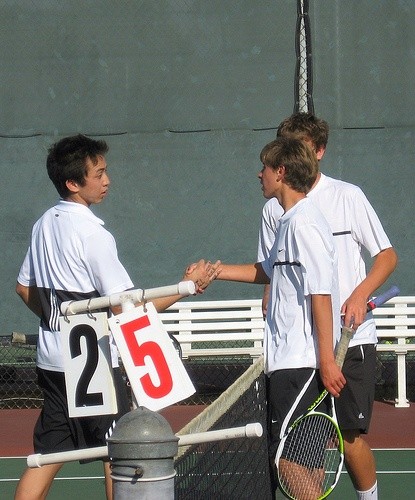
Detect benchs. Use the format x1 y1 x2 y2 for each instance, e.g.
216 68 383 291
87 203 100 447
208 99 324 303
157 296 415 408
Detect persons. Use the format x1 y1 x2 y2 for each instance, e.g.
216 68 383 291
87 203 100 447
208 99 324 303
262 112 399 500
186 138 347 500
14 133 223 500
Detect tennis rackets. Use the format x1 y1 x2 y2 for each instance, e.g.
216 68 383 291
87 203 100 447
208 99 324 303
276 285 399 500
12 332 183 362
